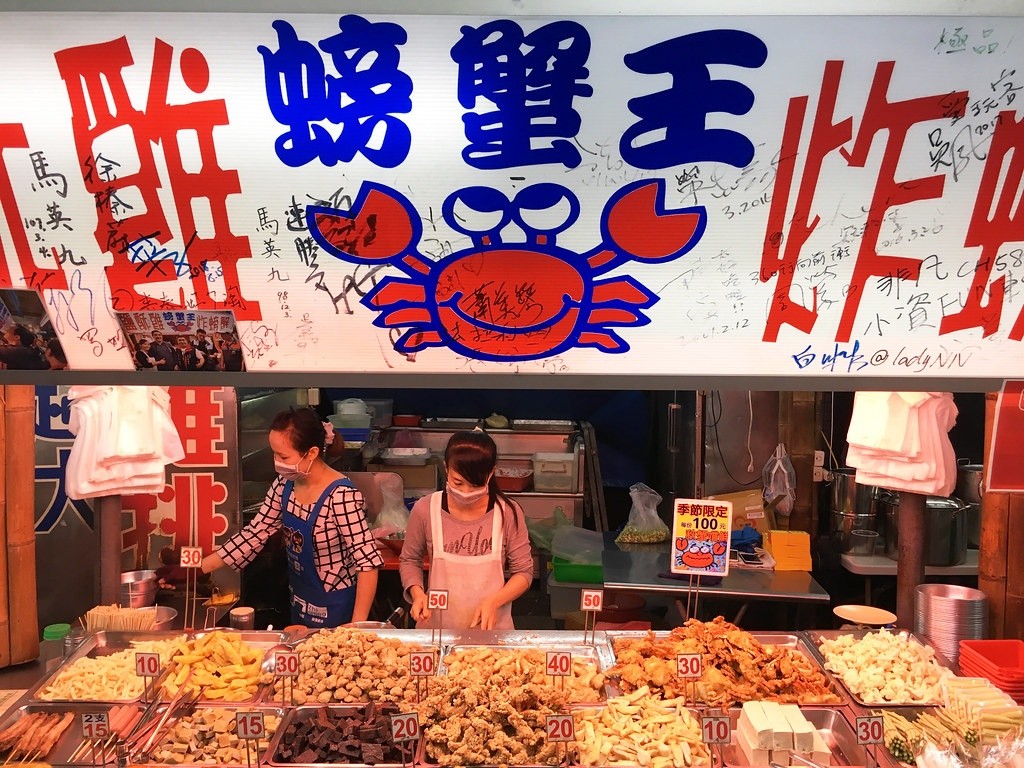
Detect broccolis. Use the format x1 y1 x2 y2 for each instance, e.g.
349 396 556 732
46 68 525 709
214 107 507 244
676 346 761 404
818 626 946 703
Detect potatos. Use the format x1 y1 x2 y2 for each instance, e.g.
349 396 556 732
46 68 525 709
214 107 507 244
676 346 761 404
160 629 268 702
574 684 711 768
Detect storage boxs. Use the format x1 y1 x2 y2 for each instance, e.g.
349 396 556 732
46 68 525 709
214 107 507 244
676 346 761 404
565 611 598 631
367 461 439 499
959 638 1024 706
550 552 601 584
333 399 393 429
703 488 786 560
529 452 576 491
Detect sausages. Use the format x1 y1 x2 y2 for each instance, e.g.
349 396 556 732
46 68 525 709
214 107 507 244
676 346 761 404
107 706 143 739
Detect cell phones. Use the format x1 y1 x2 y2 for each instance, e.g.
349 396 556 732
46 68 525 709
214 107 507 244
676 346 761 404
729 549 739 562
739 552 763 565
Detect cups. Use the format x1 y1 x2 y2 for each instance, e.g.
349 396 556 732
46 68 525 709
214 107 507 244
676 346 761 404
230 606 255 630
851 529 879 565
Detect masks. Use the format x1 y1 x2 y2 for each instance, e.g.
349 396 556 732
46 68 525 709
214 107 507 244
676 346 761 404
446 473 492 508
273 450 314 483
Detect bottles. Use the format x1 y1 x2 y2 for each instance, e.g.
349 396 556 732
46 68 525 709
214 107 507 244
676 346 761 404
39 623 85 675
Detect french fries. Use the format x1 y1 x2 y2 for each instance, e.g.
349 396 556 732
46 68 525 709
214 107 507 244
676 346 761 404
40 632 189 699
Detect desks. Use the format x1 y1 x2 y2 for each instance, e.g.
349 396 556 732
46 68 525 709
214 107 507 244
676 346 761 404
842 547 980 607
372 546 430 622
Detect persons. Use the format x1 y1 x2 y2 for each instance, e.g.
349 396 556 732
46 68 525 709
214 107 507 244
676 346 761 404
399 426 534 630
135 329 247 371
0 323 67 370
153 408 384 629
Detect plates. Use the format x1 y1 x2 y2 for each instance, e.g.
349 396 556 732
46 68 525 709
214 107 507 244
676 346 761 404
912 583 987 667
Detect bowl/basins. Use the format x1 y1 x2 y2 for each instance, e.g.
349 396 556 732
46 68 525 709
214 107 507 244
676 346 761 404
120 569 178 632
326 413 372 449
376 530 406 555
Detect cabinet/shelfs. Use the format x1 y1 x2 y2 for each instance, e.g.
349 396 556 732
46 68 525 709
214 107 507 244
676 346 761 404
119 387 310 596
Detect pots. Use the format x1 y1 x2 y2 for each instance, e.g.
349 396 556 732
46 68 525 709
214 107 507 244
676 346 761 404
337 397 367 416
826 458 985 568
